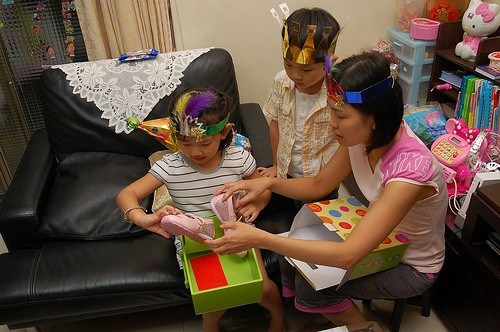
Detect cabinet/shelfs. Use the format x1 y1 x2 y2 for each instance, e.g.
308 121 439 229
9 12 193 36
429 184 500 332
389 20 500 121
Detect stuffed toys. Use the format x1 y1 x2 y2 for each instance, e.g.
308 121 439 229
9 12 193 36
455 0 500 62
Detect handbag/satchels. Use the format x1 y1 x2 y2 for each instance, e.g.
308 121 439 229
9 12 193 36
404 100 449 147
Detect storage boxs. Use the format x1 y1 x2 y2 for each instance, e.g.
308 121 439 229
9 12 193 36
277 196 414 290
181 215 264 315
410 18 440 40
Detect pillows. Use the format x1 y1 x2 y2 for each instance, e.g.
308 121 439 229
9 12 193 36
36 153 153 247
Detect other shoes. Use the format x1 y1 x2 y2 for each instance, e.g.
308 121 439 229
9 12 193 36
211 193 248 257
160 212 216 244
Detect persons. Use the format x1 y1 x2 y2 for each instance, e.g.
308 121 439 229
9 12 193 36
116 86 286 332
257 7 348 212
204 40 448 332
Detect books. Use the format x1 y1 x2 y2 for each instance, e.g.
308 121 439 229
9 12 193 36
454 76 500 133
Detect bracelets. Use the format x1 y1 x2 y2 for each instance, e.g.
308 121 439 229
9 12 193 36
124 206 147 223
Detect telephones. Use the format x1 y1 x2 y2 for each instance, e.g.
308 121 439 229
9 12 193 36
439 161 457 183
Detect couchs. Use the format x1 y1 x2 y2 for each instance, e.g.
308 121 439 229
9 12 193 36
0 48 280 332
